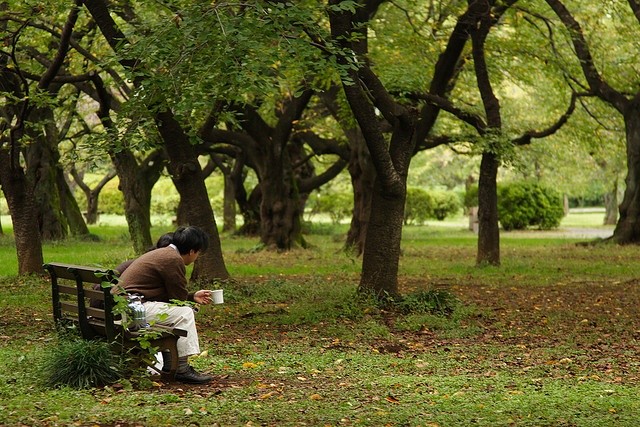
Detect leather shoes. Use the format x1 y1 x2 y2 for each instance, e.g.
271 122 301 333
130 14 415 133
161 365 214 384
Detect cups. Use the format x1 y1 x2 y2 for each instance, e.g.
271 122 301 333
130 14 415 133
206 288 225 304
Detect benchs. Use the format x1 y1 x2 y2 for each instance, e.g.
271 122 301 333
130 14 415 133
42 261 188 381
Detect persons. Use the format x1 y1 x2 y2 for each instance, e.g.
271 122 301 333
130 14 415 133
108 225 213 384
89 232 174 327
